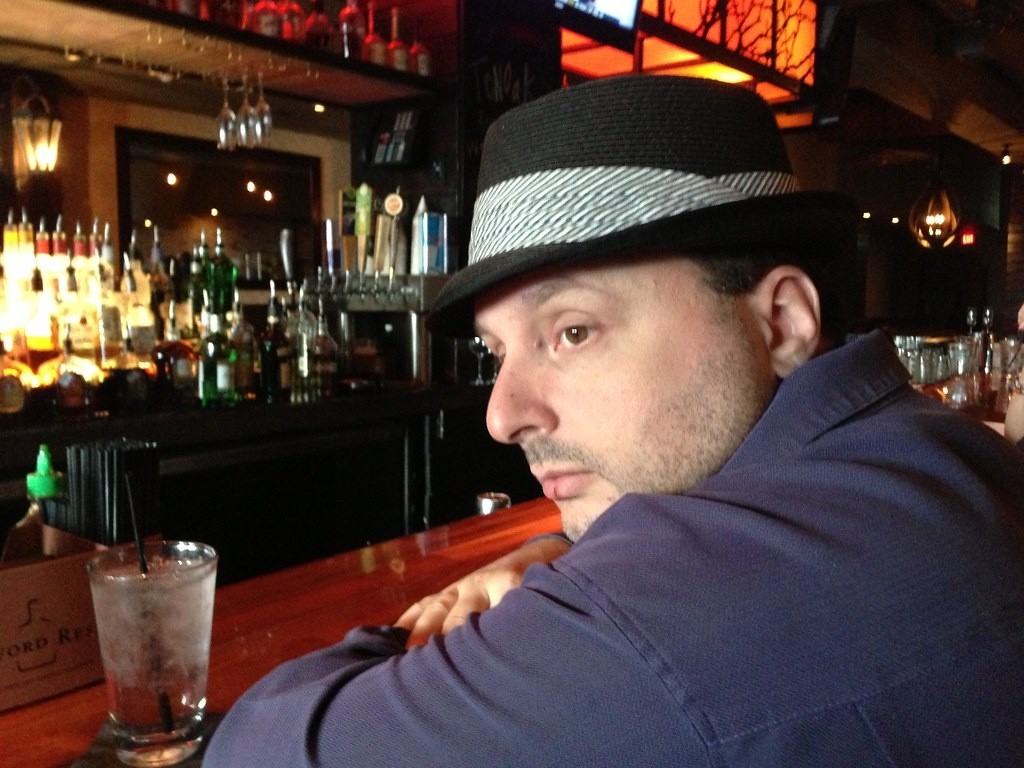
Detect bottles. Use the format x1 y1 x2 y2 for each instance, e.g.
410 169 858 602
339 0 367 58
892 334 971 386
0 208 162 354
408 30 432 77
362 2 387 65
187 227 338 408
388 7 408 70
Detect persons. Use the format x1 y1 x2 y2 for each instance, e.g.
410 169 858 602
202 77 1022 765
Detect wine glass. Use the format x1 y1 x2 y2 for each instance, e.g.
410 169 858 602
237 93 262 148
256 87 273 137
216 88 238 150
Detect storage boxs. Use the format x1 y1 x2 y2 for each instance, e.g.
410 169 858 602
0 515 123 712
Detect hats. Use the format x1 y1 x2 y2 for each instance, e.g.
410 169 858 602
424 77 861 341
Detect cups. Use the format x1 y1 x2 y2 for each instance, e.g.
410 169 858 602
85 541 219 767
